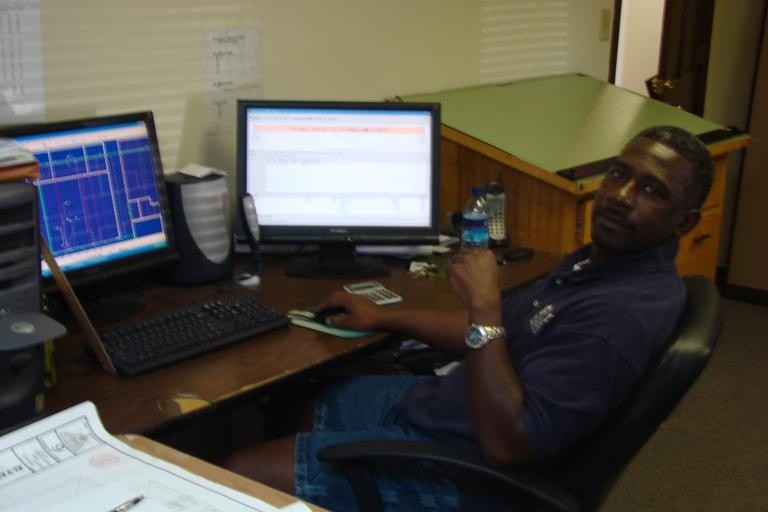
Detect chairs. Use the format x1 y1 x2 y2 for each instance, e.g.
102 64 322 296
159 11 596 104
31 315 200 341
317 272 725 512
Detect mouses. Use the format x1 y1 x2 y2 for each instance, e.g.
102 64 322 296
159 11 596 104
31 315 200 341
315 306 343 325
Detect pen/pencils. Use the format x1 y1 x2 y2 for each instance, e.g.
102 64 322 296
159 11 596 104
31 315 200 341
112 494 144 512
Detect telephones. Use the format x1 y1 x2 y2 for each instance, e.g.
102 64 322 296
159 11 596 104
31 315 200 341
483 176 512 248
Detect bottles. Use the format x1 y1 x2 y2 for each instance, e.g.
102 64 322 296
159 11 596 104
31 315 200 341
461 186 489 251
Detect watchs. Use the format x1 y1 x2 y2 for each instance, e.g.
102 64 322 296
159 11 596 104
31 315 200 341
463 322 506 349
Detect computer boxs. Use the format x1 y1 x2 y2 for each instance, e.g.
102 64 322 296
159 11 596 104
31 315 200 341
0 183 65 433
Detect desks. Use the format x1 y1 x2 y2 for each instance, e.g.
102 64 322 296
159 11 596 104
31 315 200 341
0 427 335 511
382 72 752 285
46 231 565 437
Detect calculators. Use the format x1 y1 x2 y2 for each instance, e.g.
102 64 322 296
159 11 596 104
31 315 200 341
342 281 403 306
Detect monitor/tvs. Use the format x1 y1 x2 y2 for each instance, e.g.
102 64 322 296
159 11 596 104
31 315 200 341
237 99 442 279
0 111 178 321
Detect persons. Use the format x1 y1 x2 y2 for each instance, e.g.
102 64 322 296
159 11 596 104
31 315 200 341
218 125 714 512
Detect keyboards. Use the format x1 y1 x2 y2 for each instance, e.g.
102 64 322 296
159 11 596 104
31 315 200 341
84 296 290 374
